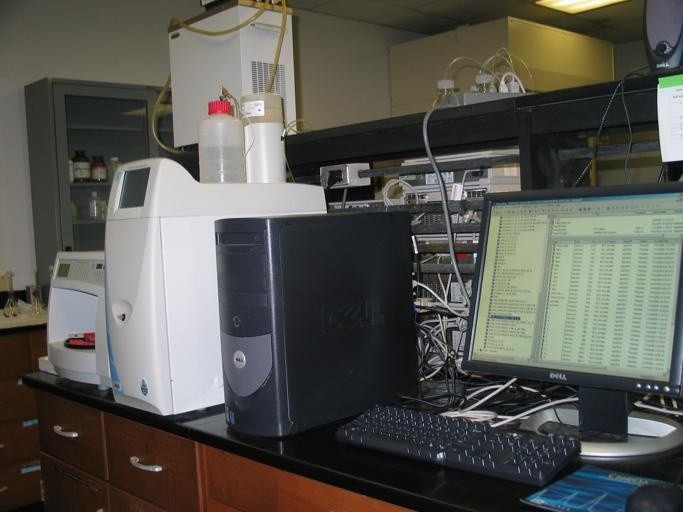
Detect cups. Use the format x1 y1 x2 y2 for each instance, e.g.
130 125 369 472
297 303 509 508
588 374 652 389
28 296 41 318
25 284 42 304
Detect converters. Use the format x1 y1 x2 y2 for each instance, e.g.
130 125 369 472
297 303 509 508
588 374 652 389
506 82 519 93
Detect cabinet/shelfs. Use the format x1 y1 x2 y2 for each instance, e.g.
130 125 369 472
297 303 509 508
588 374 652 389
39 386 413 509
19 85 169 305
1 320 46 510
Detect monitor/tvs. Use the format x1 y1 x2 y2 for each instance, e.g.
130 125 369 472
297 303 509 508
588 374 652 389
460 182 682 458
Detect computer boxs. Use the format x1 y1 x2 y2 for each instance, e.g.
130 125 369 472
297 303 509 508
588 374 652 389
214 208 418 436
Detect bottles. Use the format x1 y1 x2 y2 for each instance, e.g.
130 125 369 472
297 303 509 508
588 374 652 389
68 149 122 185
2 272 22 318
87 192 101 218
198 100 248 184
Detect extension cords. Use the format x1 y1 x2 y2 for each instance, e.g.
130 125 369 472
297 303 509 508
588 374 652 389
446 92 538 106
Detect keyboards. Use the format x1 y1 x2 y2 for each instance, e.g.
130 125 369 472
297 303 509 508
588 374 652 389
336 405 579 486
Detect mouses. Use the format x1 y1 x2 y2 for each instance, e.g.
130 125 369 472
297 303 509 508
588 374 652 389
625 485 682 510
